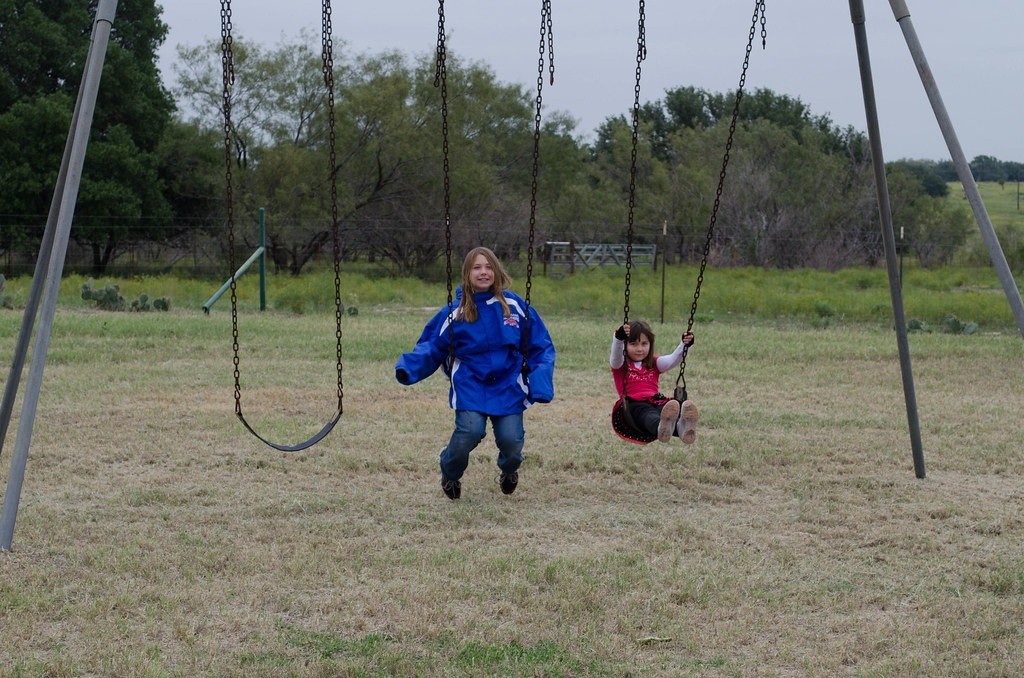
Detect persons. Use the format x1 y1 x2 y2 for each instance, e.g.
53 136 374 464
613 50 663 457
611 320 698 447
393 246 557 501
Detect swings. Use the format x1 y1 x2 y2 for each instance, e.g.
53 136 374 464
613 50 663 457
431 0 554 386
610 0 766 446
216 1 347 452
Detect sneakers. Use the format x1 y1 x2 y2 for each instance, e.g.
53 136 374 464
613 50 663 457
440 475 461 499
494 471 519 494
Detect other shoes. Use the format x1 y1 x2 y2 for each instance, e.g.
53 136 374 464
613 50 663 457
655 399 680 443
676 399 698 444
